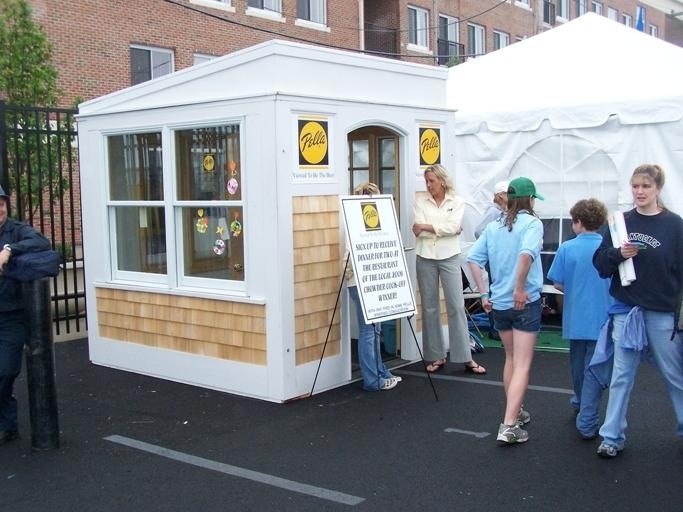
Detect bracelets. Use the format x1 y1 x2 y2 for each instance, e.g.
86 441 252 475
480 293 490 300
3 243 12 252
480 289 488 294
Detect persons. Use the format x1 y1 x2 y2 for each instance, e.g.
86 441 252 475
411 163 487 374
0 188 50 449
591 163 683 458
474 179 511 340
545 198 614 439
342 182 402 393
464 176 546 446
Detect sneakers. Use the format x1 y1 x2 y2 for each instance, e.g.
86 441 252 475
391 376 402 382
517 408 530 425
597 444 617 457
497 423 529 443
381 378 397 390
0 431 18 445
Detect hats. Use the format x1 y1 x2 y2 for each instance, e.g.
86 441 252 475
507 177 545 201
493 181 509 194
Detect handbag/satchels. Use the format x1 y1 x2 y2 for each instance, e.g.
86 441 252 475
5 250 60 283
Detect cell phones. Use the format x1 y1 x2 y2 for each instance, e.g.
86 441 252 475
629 242 648 250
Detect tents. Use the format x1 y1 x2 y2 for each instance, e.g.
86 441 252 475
444 12 683 298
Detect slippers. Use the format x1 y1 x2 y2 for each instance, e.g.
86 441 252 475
426 363 443 373
465 364 486 374
